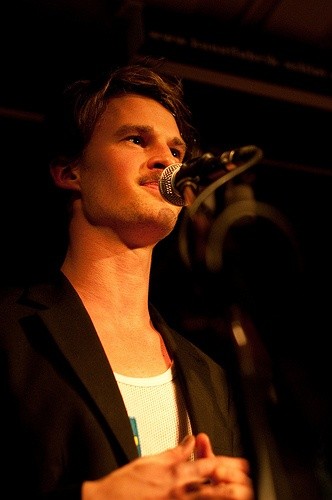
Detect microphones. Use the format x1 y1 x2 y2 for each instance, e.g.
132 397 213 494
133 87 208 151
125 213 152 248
159 146 257 206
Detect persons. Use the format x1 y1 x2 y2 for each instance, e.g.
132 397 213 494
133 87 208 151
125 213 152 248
0 62 255 500
